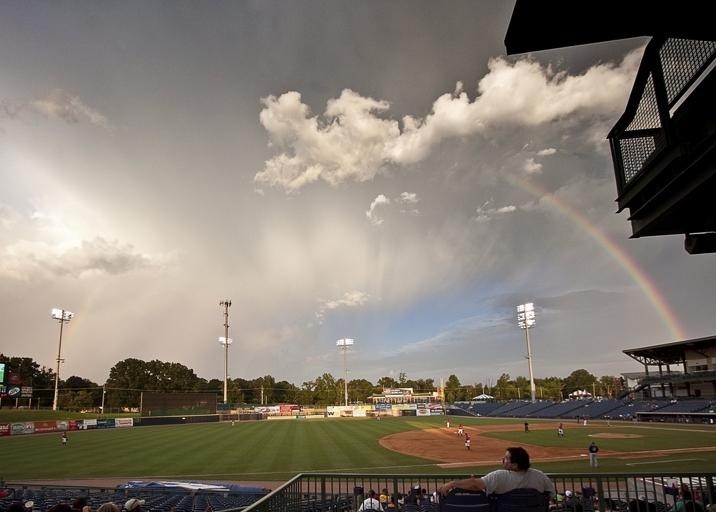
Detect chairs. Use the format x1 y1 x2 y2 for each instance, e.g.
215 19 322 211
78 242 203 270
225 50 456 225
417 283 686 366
0 480 716 512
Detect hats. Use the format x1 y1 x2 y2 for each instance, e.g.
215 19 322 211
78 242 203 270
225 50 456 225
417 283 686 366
552 490 574 504
123 497 146 512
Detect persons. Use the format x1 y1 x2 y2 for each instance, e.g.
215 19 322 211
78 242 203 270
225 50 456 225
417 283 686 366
549 476 716 512
62 435 66 447
231 418 234 427
524 420 529 432
457 423 463 436
557 423 564 440
463 431 472 450
295 413 298 423
0 477 146 512
603 399 715 425
582 414 587 426
589 441 600 467
439 446 557 501
63 431 68 441
353 485 452 512
446 419 449 429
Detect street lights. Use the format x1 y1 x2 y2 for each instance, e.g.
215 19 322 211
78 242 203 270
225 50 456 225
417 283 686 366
335 337 354 407
516 302 536 403
219 337 233 404
51 308 74 411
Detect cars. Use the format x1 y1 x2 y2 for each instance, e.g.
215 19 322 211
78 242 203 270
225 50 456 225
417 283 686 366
121 407 139 413
81 407 101 413
